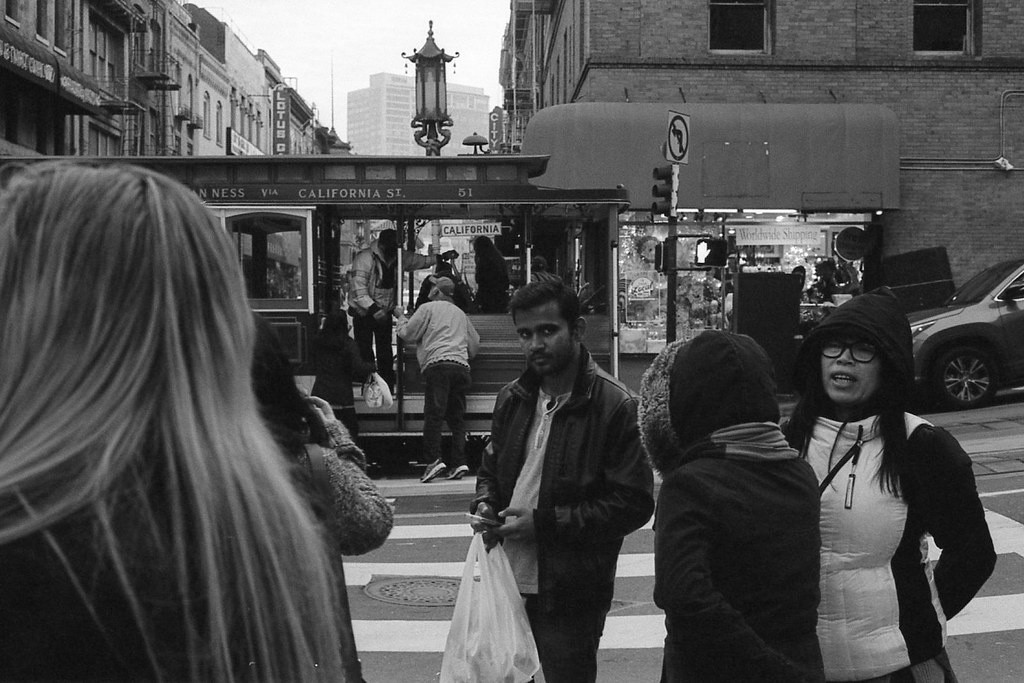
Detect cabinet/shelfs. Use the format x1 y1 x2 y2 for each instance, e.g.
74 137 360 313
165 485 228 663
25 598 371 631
626 270 661 325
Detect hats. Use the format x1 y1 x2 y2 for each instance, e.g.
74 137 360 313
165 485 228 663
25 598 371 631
429 276 455 297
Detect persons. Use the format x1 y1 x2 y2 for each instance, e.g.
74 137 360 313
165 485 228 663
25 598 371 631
394 276 480 482
414 235 567 311
638 330 827 683
0 165 396 683
780 287 996 683
468 277 654 683
346 227 459 392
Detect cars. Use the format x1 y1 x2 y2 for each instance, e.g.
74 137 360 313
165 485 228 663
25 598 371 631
905 256 1024 412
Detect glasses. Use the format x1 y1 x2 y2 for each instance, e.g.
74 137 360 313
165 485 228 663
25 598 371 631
820 337 883 363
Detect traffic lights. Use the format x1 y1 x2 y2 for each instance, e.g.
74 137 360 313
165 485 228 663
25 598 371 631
651 165 671 216
694 238 729 270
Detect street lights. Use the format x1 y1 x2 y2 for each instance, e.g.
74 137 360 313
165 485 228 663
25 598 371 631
402 20 460 274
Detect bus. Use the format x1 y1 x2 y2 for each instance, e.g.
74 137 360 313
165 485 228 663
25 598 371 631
0 155 618 465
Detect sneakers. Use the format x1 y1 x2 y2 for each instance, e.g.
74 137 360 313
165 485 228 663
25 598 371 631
446 465 469 480
421 459 446 483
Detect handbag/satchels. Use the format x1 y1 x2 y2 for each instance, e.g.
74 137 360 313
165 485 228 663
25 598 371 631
438 530 540 683
364 373 383 408
373 372 394 410
